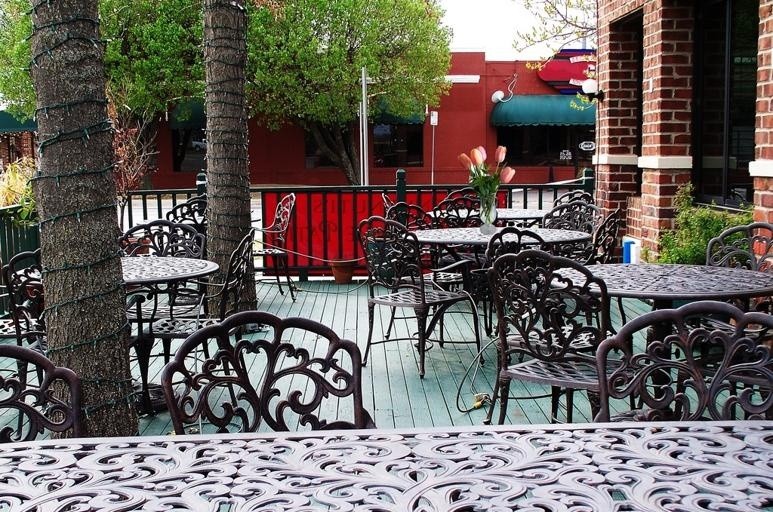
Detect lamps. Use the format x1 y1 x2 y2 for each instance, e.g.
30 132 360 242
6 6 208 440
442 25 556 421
489 72 518 104
581 78 604 105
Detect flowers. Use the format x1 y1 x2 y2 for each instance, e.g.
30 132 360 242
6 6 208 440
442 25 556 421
457 145 515 225
